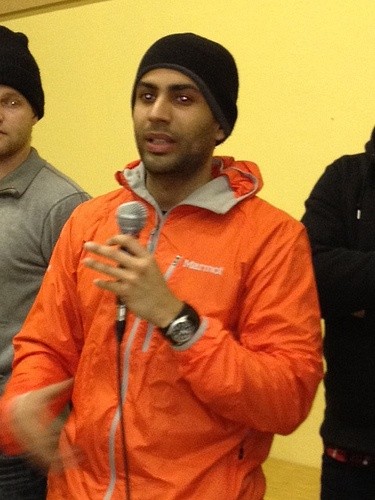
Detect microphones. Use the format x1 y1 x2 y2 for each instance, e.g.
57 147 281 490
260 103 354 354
115 201 148 340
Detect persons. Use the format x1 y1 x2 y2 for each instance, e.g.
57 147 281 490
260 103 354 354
302 126 375 500
0 25 92 500
0 33 323 500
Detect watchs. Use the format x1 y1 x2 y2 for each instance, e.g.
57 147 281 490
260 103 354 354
159 303 199 346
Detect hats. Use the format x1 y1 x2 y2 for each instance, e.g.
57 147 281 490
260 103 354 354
131 32 239 144
0 26 44 120
